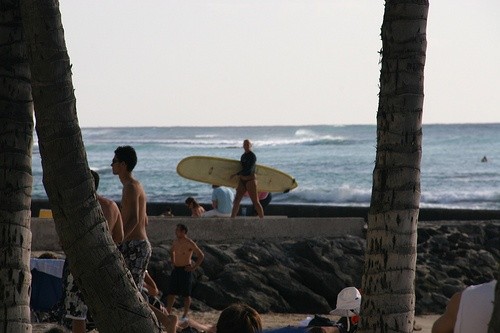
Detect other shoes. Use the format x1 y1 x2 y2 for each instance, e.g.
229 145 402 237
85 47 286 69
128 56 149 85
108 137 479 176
180 316 187 321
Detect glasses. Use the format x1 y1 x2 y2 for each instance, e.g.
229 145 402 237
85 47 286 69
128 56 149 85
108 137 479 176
112 159 121 163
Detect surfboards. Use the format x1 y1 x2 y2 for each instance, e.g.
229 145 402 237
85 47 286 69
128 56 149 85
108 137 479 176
176 155 297 193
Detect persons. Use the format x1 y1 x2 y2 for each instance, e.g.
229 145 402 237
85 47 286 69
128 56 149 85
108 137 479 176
166 223 204 318
65 169 124 333
177 318 217 333
244 191 273 214
109 145 177 333
37 252 58 260
229 140 265 220
431 279 500 333
184 197 205 217
335 309 360 333
141 268 215 333
161 206 175 216
215 303 262 333
202 184 237 218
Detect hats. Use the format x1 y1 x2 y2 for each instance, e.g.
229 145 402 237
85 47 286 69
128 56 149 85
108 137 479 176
329 287 362 317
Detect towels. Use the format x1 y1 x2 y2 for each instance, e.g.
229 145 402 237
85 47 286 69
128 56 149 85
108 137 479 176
29 257 64 281
